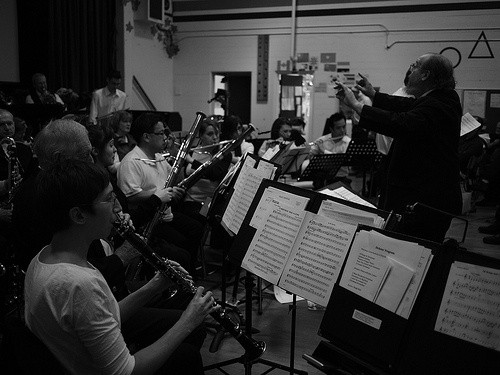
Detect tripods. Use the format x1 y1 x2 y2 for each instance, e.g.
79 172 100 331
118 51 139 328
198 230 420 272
193 222 309 375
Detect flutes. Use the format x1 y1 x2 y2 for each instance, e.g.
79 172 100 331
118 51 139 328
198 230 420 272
113 220 267 365
308 135 344 146
190 139 233 151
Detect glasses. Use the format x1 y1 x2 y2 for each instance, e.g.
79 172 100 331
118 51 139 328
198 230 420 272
150 130 165 136
73 190 115 205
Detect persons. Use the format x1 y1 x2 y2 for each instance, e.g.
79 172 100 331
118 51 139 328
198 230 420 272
333 53 464 248
115 112 208 284
9 116 208 343
0 69 415 271
24 154 222 375
459 116 500 244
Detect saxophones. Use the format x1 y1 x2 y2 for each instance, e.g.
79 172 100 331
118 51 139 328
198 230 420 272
0 135 19 209
